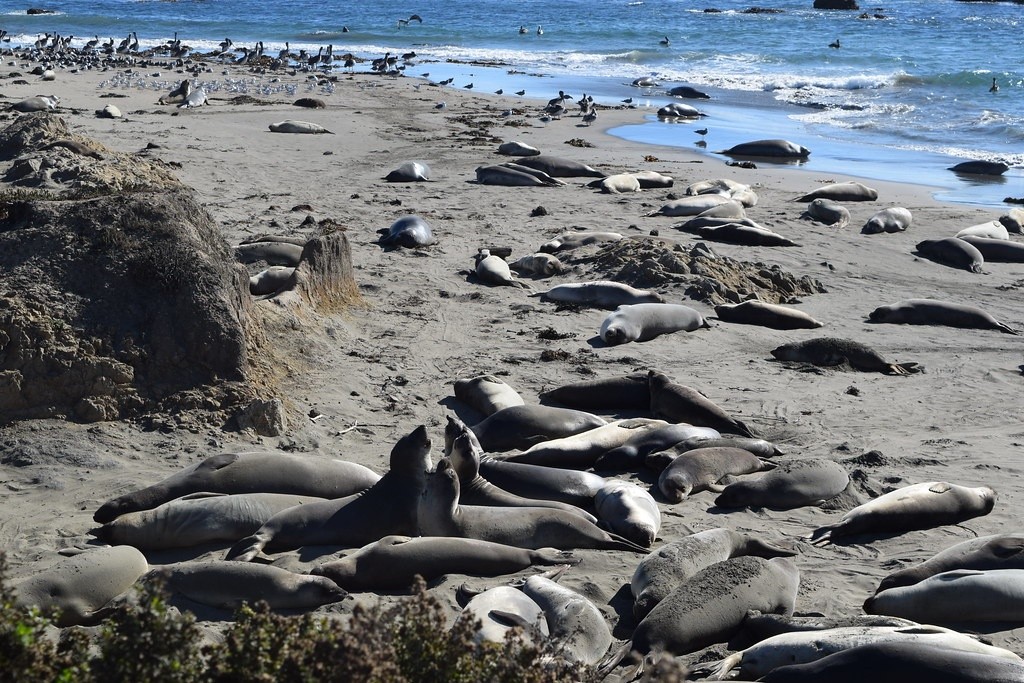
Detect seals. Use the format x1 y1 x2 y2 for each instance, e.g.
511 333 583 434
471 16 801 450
3 78 1024 683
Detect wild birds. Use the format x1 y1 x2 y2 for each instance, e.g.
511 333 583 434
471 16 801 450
0 13 1006 171
